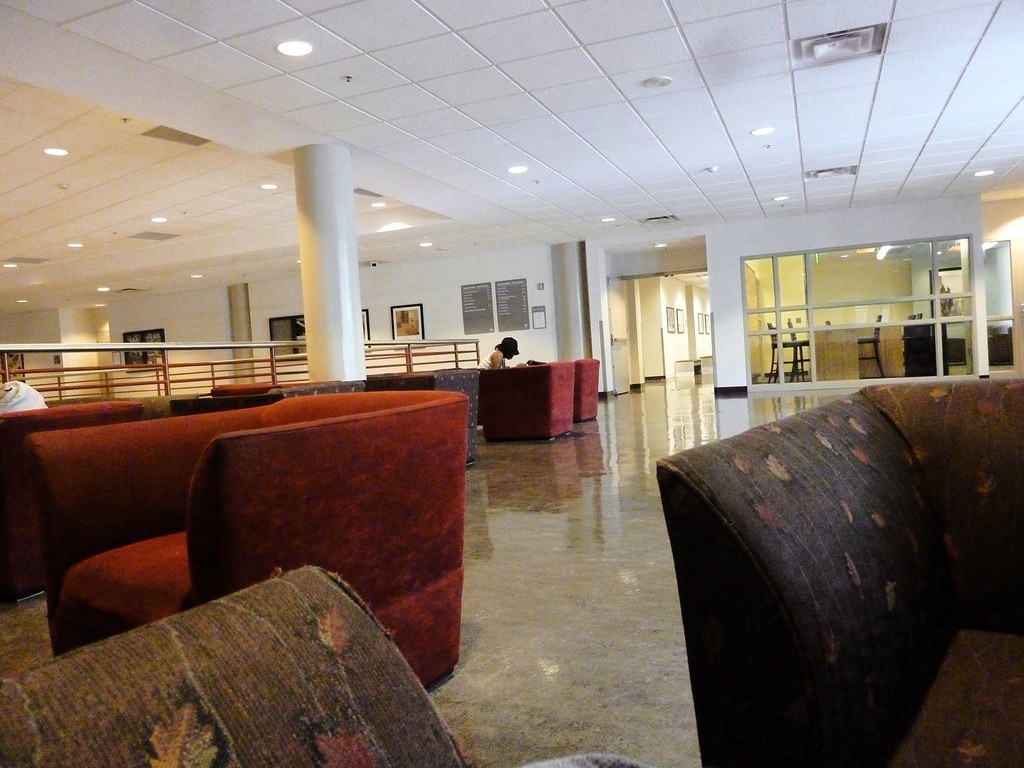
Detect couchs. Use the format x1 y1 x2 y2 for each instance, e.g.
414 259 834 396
0 399 149 609
211 359 600 470
656 380 1024 768
0 566 471 768
24 390 469 690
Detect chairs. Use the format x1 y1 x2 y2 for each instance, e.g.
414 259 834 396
767 319 810 384
858 314 885 378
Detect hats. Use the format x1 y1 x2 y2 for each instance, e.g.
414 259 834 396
494 336 519 355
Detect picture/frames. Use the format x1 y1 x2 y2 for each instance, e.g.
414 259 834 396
676 309 684 333
391 303 425 349
705 314 711 334
698 313 704 334
362 309 371 348
666 307 675 333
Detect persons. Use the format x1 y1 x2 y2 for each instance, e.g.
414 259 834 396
0 380 49 415
477 337 519 369
